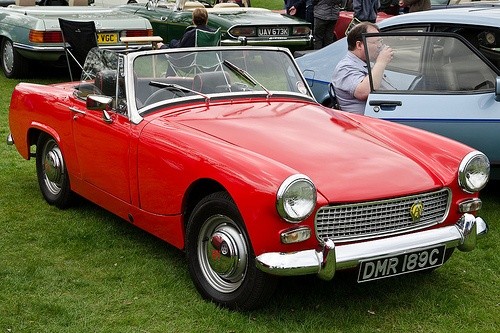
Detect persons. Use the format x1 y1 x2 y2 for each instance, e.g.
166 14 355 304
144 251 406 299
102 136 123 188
157 8 218 78
284 0 431 51
332 21 393 116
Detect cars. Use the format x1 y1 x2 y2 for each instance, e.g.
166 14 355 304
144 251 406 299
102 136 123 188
287 5 500 184
0 0 500 80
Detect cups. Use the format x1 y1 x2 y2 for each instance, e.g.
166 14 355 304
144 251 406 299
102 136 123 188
378 44 386 52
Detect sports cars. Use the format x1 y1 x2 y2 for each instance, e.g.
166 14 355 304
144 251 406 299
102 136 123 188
6 42 492 313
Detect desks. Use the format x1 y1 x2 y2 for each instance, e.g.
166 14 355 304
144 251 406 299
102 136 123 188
120 35 162 78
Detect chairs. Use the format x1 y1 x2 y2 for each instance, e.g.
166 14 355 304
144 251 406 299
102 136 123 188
437 27 496 94
186 70 232 96
68 0 89 6
164 25 226 78
94 69 145 115
184 1 205 10
58 17 139 83
212 2 241 9
7 0 39 8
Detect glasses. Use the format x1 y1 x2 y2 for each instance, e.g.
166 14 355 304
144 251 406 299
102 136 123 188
366 39 383 46
